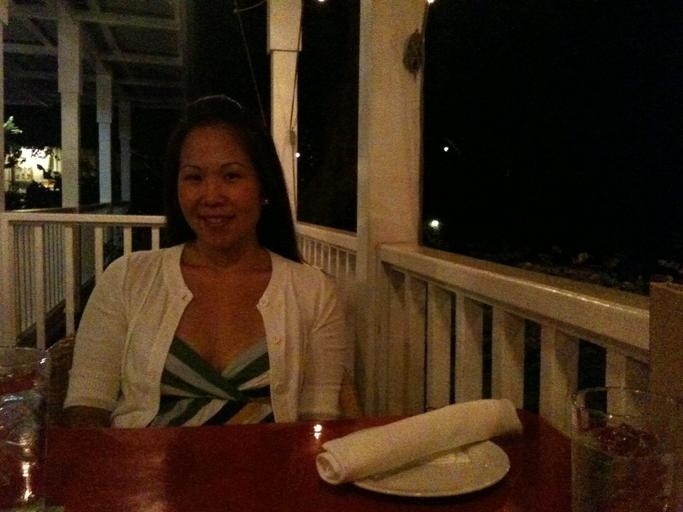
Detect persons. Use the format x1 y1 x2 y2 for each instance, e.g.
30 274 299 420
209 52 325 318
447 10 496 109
64 94 360 430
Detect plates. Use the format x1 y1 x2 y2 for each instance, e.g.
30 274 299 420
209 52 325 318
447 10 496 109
351 439 512 497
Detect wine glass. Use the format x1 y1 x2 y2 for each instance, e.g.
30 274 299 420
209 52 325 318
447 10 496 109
0 347 52 512
570 387 677 512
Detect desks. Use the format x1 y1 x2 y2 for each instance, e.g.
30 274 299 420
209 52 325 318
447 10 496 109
0 409 683 511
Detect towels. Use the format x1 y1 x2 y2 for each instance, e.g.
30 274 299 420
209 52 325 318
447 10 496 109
315 396 524 485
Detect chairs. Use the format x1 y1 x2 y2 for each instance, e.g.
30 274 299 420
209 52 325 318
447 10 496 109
41 335 78 427
